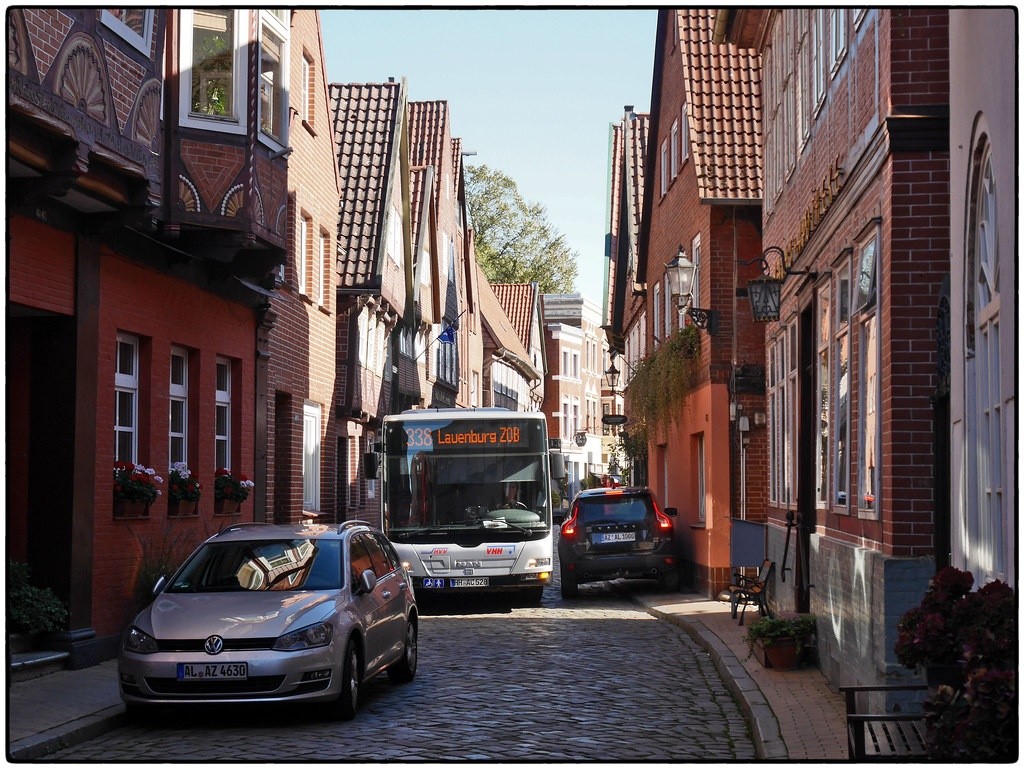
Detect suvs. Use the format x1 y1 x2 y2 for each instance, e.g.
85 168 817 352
558 485 678 602
117 517 418 723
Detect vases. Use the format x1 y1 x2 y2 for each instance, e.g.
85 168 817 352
113 497 150 517
214 498 243 514
168 499 198 517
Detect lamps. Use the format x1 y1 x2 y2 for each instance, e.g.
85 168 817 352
746 246 806 322
661 243 719 335
604 362 623 397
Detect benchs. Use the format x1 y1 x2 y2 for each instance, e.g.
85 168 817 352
728 559 775 626
840 659 974 759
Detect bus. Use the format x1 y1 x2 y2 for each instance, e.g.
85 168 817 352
364 406 564 607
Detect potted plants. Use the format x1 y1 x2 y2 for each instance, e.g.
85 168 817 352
744 614 818 669
7 558 75 652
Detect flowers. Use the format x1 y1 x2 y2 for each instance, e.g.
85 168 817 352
168 461 202 507
897 564 1018 759
112 460 162 504
214 467 254 502
